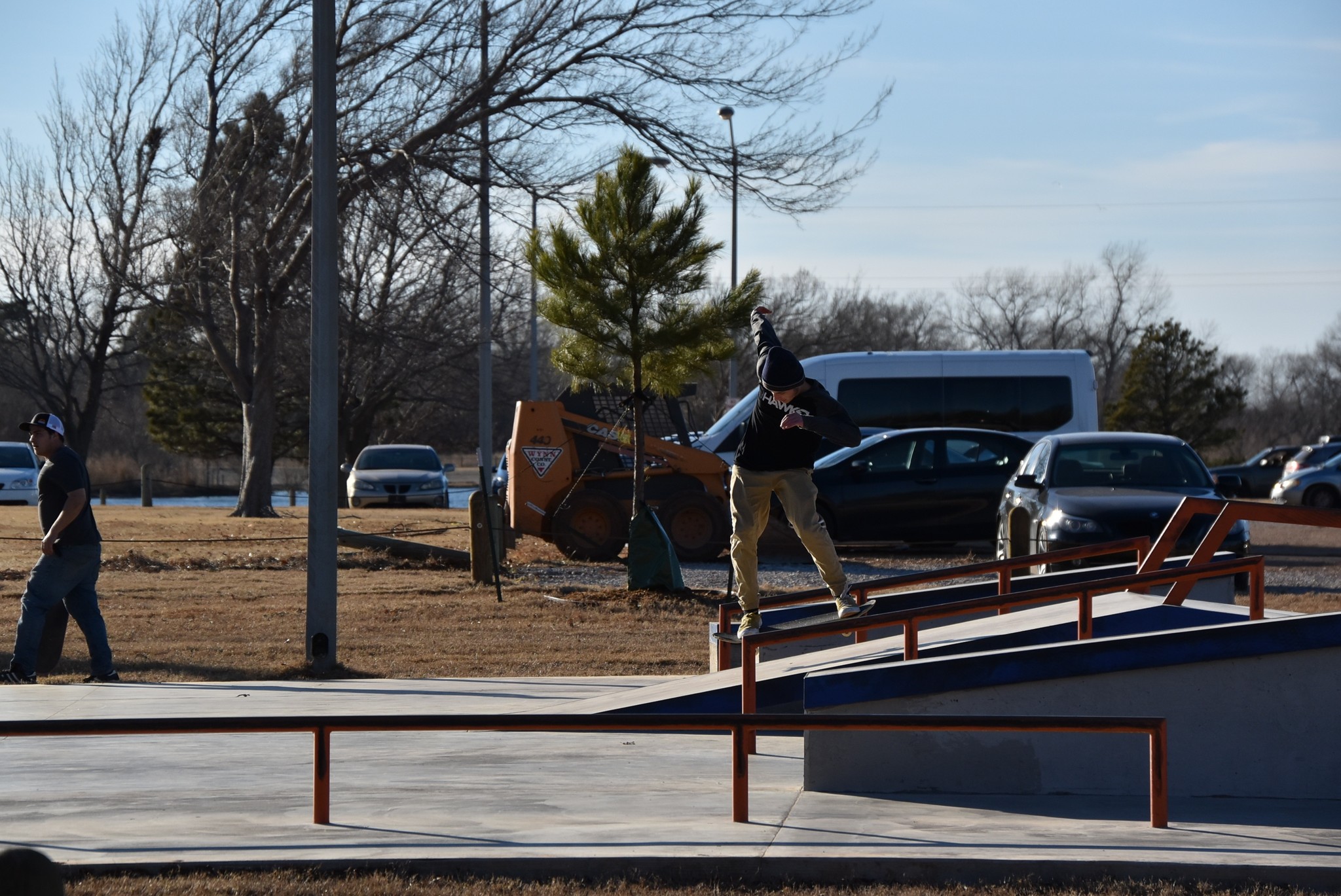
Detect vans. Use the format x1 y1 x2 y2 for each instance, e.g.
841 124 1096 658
692 350 1098 475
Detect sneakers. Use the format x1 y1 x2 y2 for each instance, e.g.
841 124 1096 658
737 608 762 639
834 579 861 619
83 669 120 684
0 670 37 683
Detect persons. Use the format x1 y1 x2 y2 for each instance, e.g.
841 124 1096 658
0 413 121 684
730 306 861 638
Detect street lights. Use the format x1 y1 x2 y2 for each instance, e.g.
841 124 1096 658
719 106 738 284
530 156 673 403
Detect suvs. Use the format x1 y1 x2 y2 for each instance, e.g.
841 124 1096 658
1209 445 1303 497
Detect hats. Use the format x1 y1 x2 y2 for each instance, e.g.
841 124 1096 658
761 346 804 391
18 412 64 436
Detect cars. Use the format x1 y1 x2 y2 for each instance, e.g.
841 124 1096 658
340 445 455 510
767 427 1037 554
990 432 1250 595
0 442 47 507
491 430 708 500
1271 443 1341 510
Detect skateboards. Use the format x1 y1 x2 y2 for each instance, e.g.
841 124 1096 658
35 598 70 674
711 599 876 654
